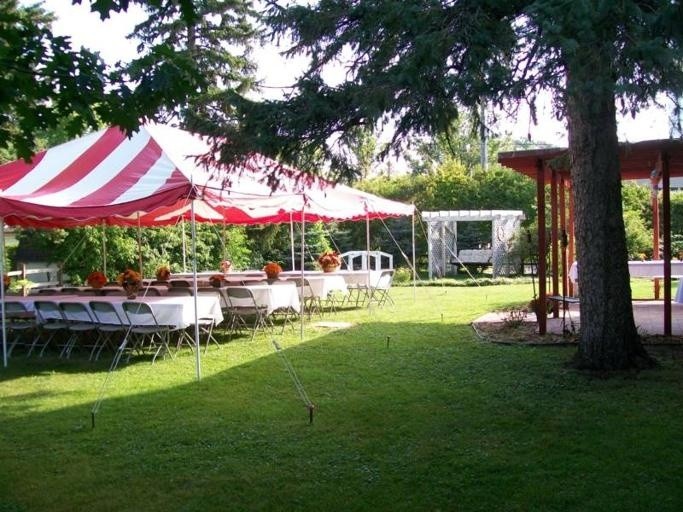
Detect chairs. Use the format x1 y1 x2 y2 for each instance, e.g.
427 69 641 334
451 249 492 265
1 267 396 368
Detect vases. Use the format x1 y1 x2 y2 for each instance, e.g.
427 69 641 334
320 264 338 272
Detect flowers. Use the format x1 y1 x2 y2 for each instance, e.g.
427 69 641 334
264 263 282 274
221 261 231 270
318 251 341 266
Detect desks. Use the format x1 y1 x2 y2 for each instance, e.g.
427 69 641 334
567 261 682 282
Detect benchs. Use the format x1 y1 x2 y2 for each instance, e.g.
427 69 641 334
548 293 580 308
